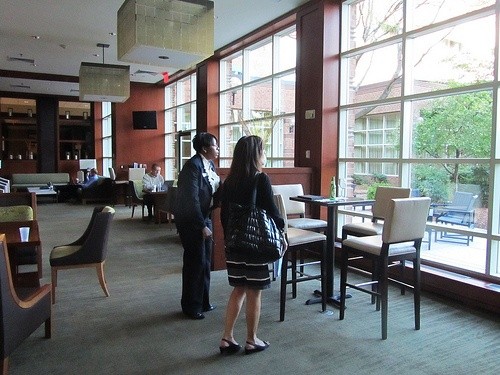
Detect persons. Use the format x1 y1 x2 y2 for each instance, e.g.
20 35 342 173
142 164 167 223
220 135 288 354
175 133 224 320
63 169 99 201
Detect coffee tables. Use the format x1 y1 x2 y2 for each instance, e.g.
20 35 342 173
28 187 59 207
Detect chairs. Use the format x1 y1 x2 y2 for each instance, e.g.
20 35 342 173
272 184 478 342
0 167 178 375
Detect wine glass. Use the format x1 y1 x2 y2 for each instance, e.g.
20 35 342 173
338 179 347 199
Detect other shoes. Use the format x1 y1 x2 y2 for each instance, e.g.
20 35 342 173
203 305 215 312
245 340 269 354
148 214 152 219
219 338 241 353
182 310 205 319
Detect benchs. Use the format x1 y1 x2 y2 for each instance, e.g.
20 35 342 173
80 176 112 205
11 173 70 192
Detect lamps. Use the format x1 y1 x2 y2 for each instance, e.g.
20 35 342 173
116 0 215 70
78 43 131 104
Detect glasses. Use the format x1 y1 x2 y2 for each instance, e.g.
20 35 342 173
205 143 218 149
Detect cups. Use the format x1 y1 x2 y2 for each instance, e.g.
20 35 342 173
158 183 161 192
19 227 30 241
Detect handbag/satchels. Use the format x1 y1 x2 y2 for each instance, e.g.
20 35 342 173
245 172 288 262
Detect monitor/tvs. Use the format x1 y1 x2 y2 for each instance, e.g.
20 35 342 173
132 111 158 130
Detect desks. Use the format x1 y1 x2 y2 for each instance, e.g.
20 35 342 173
429 204 450 207
288 195 376 310
142 189 169 225
0 220 42 289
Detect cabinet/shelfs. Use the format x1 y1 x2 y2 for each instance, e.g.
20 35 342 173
2 117 38 159
59 119 93 161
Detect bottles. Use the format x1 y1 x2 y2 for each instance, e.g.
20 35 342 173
329 177 336 200
